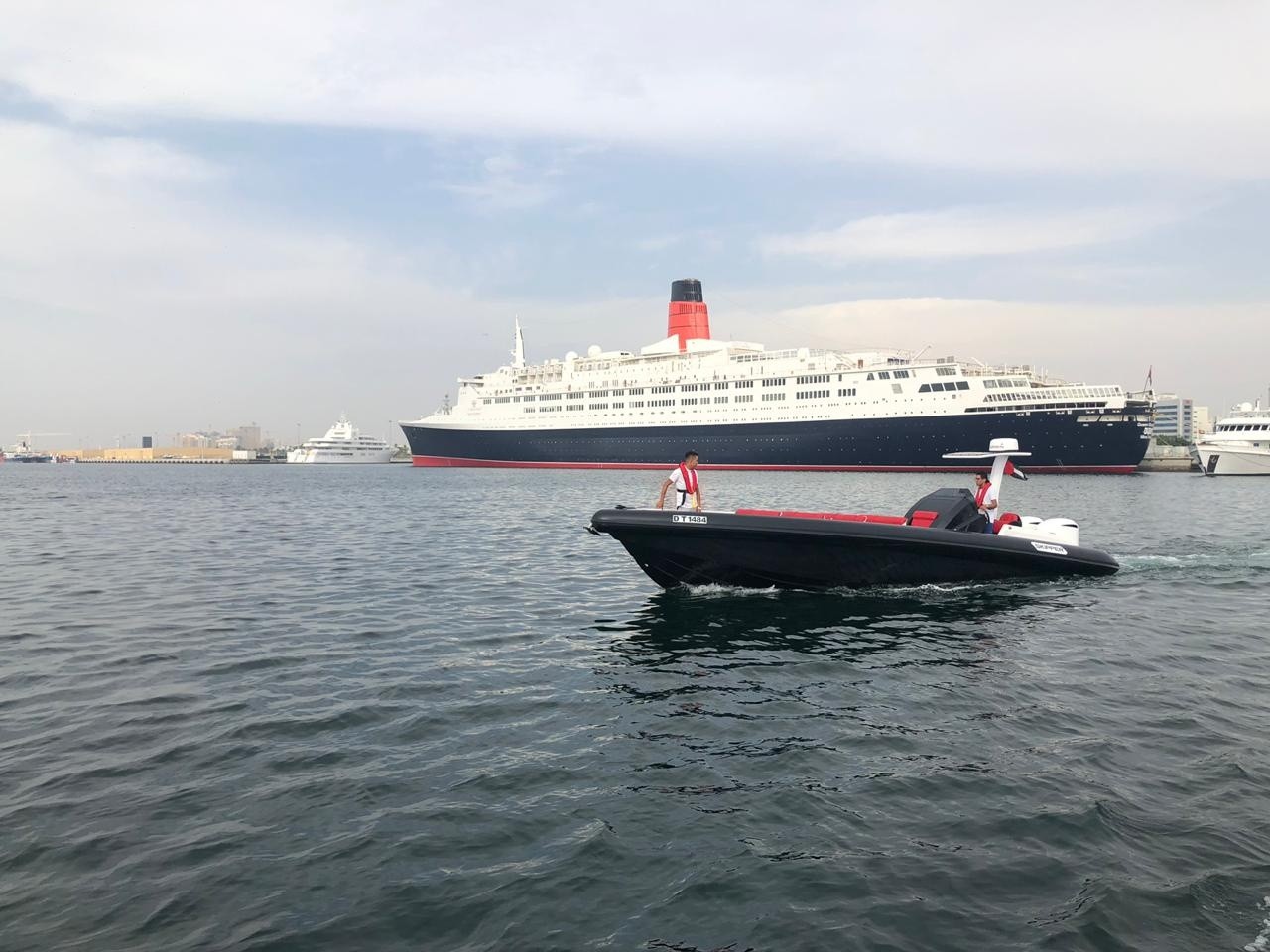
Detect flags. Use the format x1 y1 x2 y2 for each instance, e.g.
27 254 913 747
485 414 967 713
1148 369 1152 385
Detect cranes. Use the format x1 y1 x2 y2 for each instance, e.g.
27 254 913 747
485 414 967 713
16 430 71 451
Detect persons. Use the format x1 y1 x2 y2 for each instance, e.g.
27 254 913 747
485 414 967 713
655 451 702 511
974 472 998 533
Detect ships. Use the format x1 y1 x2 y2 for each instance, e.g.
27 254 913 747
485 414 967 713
394 278 1159 477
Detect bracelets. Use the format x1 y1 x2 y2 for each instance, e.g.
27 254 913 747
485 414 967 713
697 506 701 509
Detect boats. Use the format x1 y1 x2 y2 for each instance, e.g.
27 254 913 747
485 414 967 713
284 412 400 464
582 435 1123 592
1187 389 1269 478
